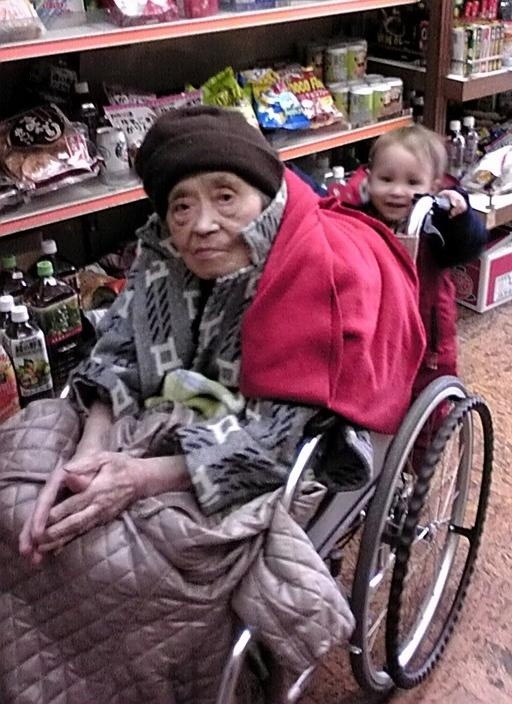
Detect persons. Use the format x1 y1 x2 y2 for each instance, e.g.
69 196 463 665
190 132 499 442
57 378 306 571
339 122 490 477
14 102 430 569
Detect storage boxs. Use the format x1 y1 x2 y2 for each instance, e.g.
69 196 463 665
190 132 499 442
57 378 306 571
451 237 512 312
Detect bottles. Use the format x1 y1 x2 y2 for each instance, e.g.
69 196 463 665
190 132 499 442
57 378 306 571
447 114 479 179
1 241 93 424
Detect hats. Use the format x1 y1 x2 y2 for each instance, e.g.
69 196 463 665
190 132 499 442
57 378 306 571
134 106 283 198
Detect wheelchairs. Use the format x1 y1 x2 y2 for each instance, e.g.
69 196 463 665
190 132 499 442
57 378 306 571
59 193 495 702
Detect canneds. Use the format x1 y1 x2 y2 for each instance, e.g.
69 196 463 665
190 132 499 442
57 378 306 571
303 38 403 127
450 0 505 77
96 125 130 185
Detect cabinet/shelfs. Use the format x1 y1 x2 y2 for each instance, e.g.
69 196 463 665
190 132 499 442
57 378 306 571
0 0 512 237
